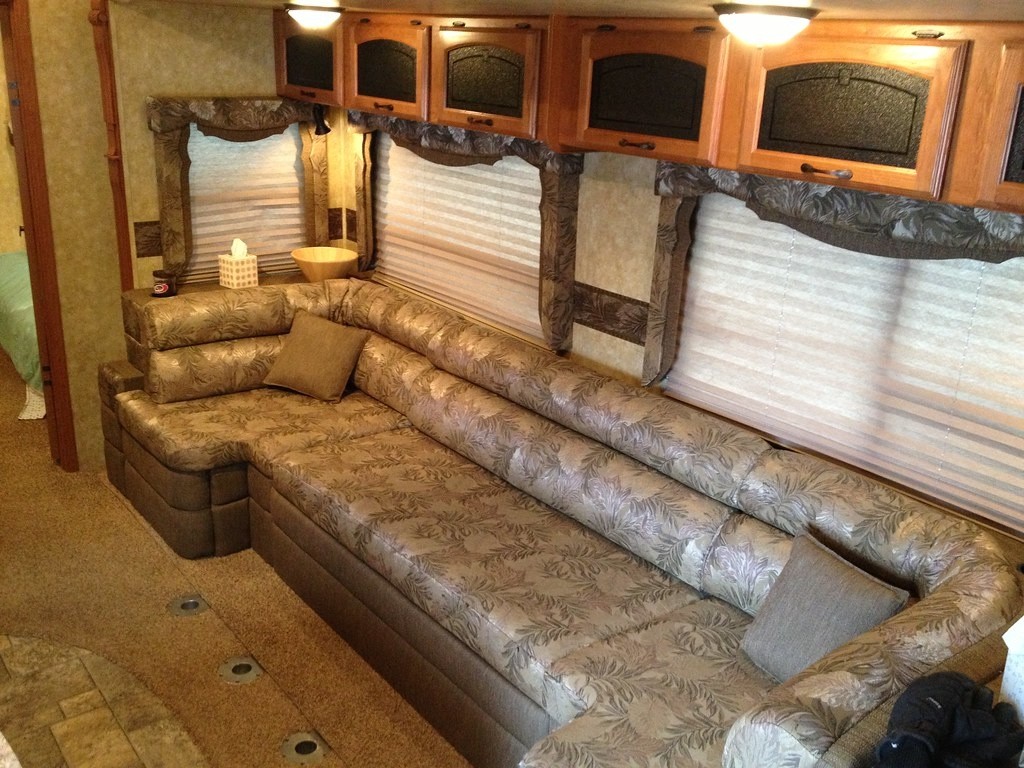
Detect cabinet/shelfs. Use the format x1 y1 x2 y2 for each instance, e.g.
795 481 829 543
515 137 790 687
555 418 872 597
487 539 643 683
272 4 1024 213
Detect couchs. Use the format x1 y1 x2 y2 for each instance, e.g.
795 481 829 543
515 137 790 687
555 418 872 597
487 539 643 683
96 272 1024 767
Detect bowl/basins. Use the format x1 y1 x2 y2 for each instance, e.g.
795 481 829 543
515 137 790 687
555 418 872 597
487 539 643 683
290 246 358 282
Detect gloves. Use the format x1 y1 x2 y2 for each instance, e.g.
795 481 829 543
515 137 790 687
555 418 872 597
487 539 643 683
874 670 1023 768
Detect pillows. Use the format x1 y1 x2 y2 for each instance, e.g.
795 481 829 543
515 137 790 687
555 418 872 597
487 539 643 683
736 531 912 686
263 308 373 405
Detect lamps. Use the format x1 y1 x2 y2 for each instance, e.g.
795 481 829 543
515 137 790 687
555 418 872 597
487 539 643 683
283 5 346 32
712 4 821 49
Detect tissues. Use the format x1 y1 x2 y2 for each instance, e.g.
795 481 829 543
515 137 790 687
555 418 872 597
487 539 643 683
218 238 259 290
996 614 1024 729
